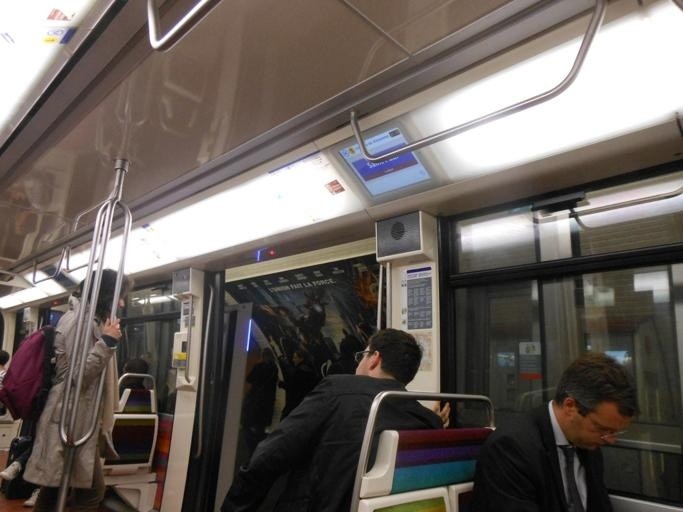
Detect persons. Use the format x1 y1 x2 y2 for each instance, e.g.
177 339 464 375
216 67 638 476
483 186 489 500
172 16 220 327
0 348 11 416
468 351 642 512
0 447 41 508
116 358 150 398
218 325 451 511
21 268 132 512
237 266 388 474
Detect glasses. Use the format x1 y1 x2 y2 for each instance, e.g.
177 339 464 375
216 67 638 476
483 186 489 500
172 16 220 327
354 349 375 364
577 404 634 444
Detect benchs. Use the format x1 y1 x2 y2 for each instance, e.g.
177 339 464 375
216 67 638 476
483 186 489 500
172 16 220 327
350 390 497 512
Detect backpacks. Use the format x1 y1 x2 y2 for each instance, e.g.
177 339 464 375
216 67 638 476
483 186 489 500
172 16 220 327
0 324 57 423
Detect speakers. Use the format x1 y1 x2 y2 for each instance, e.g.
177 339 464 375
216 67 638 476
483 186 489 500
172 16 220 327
172 266 202 301
374 211 435 263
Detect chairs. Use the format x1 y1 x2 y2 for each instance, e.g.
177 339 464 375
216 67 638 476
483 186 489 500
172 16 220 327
97 371 158 511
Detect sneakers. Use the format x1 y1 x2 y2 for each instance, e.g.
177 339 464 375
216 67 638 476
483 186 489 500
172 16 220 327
21 488 39 508
0 461 22 482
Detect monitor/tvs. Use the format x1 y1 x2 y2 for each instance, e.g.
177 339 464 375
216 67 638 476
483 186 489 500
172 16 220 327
40 266 77 289
321 116 448 207
